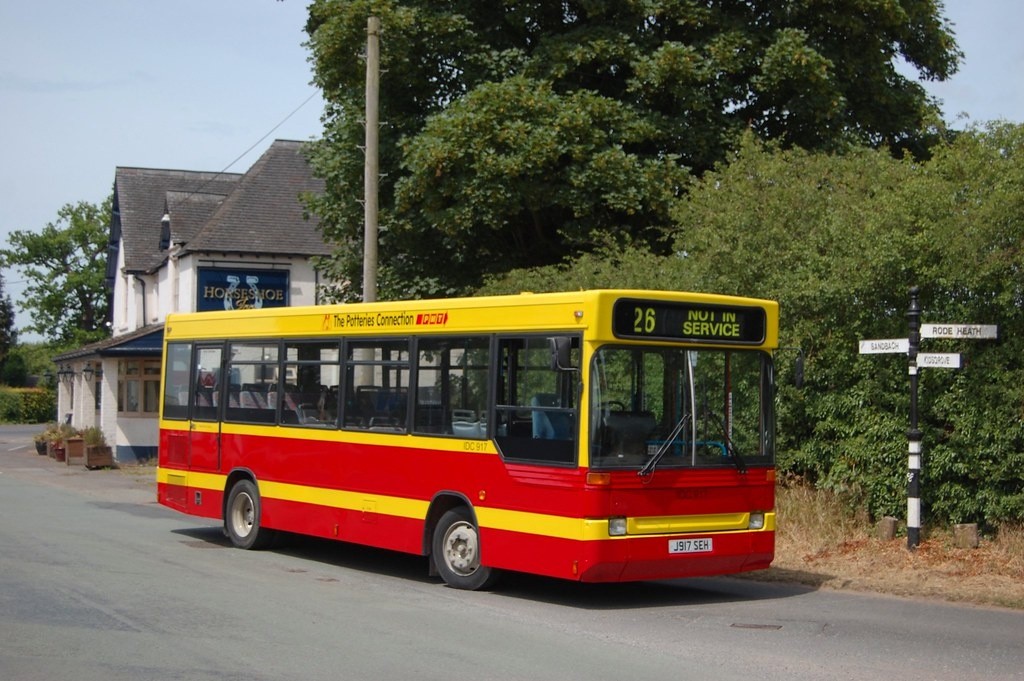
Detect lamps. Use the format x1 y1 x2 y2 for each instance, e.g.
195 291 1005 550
81 365 102 383
42 370 57 384
57 368 64 382
64 366 81 382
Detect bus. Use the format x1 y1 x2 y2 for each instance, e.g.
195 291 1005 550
157 288 807 591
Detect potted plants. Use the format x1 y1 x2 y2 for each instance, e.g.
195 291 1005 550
64 426 85 466
82 425 113 470
33 421 67 462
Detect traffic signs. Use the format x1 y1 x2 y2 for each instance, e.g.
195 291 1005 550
858 324 997 368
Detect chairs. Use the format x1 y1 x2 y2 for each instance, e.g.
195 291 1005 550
176 382 573 441
